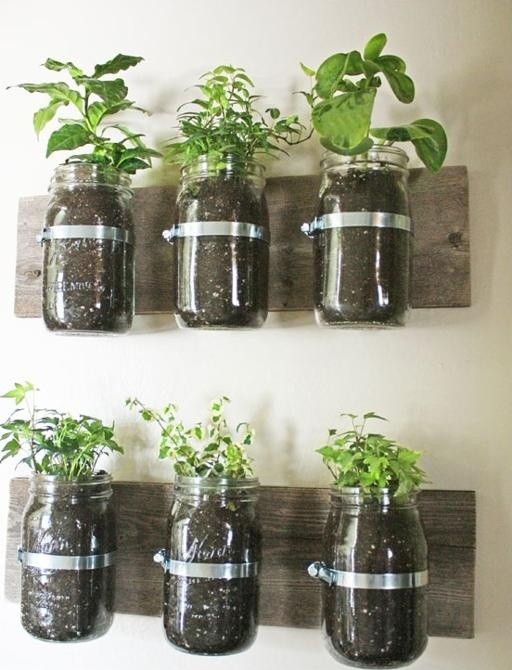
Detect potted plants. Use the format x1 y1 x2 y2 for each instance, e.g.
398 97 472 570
311 32 448 328
5 52 162 337
164 64 318 330
0 384 125 642
316 412 432 669
124 397 263 654
